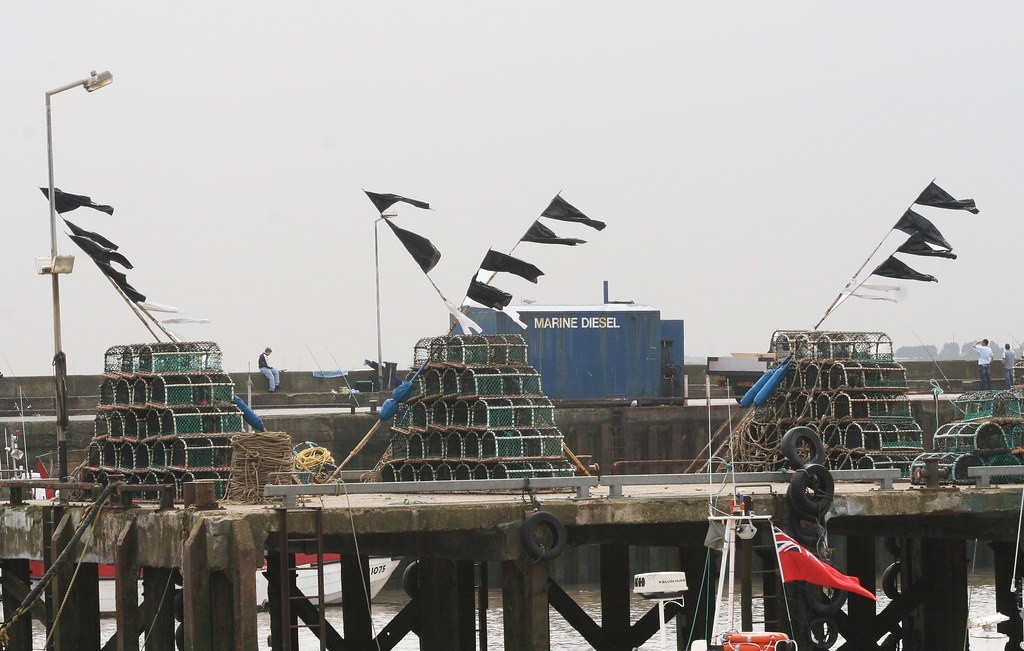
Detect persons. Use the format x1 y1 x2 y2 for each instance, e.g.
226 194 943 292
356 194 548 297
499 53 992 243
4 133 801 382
1002 344 1015 389
972 339 994 390
259 348 281 392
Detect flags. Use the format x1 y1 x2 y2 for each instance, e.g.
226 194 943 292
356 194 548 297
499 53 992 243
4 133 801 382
894 208 955 250
66 230 146 304
542 193 606 233
915 181 979 214
364 185 431 212
519 219 586 248
64 218 119 252
773 525 877 600
875 253 939 286
465 272 512 311
42 186 114 216
898 234 957 262
481 246 543 284
381 217 439 274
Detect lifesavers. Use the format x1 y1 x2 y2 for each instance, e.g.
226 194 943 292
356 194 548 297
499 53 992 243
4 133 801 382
402 561 421 599
784 463 850 651
881 536 904 637
779 424 828 468
721 630 790 651
520 510 567 563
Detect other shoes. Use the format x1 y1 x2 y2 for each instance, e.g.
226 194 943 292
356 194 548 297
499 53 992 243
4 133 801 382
269 388 276 393
275 384 280 388
